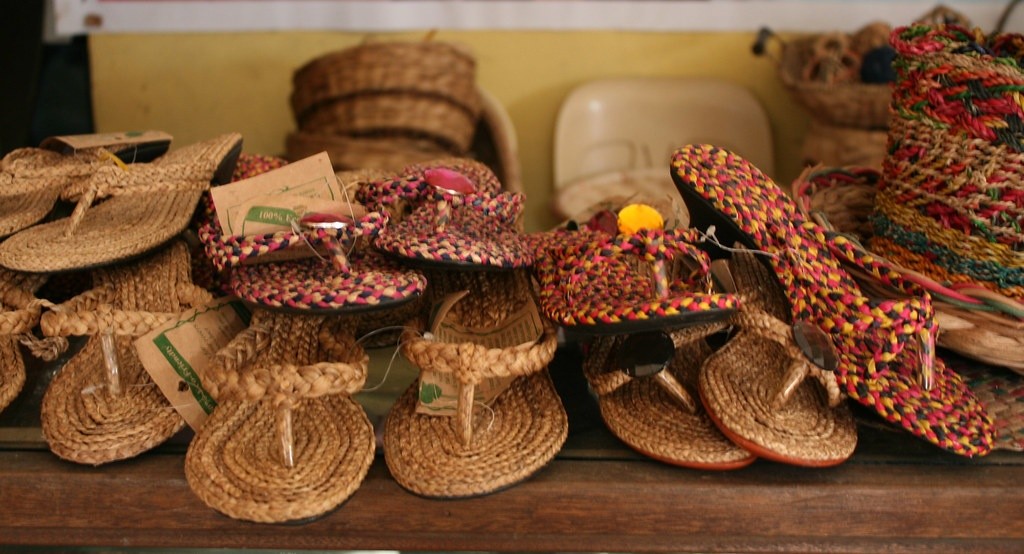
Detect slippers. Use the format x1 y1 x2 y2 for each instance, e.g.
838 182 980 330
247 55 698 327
0 127 996 525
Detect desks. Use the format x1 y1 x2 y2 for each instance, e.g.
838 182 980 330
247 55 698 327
1 368 1024 554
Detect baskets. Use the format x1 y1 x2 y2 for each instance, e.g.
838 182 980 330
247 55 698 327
287 26 479 121
799 125 886 168
286 131 449 169
790 157 1024 371
778 33 888 128
302 93 477 153
